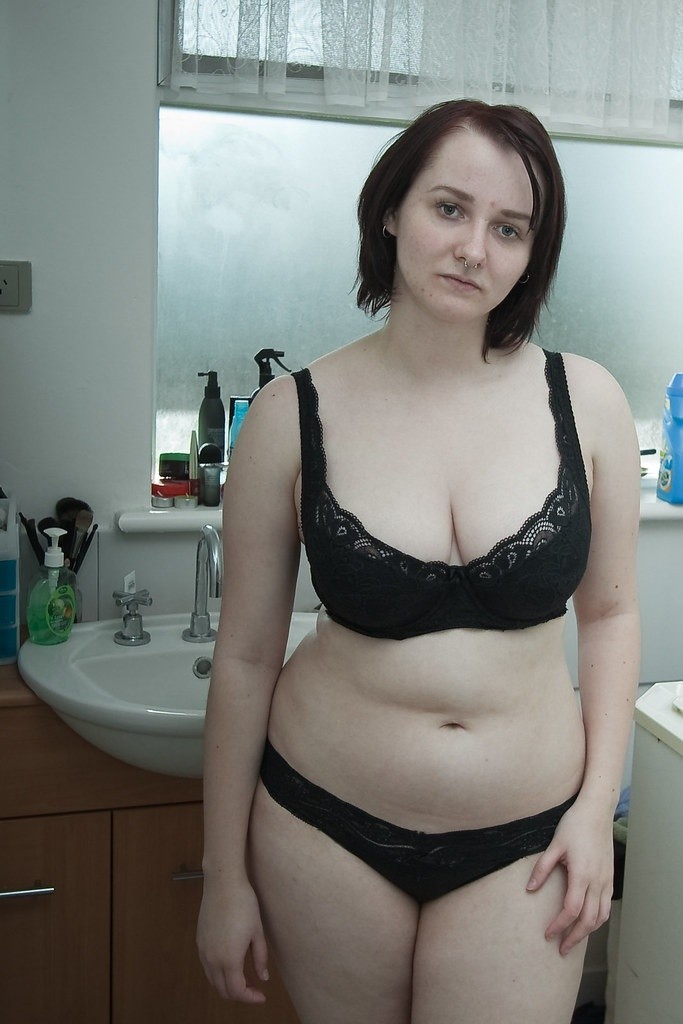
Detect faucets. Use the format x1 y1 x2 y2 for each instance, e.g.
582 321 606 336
180 524 223 642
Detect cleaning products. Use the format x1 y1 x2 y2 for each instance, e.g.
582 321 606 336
656 372 682 507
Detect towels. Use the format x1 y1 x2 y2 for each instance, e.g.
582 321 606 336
605 783 633 900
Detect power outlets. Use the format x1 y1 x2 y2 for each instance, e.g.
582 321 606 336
0 259 32 314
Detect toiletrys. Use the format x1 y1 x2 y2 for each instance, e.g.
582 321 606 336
26 527 77 646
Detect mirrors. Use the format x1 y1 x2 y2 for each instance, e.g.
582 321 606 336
151 105 683 480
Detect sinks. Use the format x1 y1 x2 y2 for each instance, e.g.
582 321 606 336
17 612 319 778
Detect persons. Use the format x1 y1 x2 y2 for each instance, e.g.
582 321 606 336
196 96 642 1023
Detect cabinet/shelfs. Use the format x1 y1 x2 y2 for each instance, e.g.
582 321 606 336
0 706 300 1024
